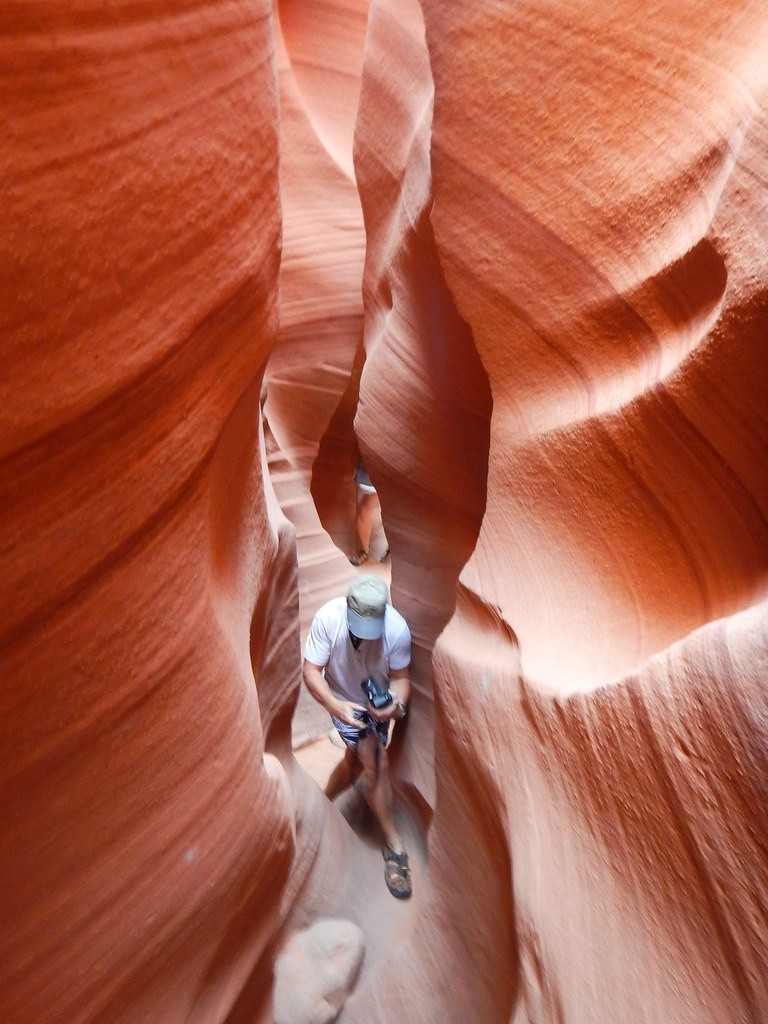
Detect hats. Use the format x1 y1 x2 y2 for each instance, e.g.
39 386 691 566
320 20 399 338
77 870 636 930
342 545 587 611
346 575 387 641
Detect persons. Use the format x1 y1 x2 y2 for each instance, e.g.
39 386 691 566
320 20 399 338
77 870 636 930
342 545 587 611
350 464 389 566
301 575 412 899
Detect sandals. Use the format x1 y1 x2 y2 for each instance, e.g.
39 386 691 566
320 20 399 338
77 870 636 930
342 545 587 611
382 845 412 896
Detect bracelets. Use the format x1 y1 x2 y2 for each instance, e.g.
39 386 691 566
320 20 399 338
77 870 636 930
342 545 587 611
393 703 406 721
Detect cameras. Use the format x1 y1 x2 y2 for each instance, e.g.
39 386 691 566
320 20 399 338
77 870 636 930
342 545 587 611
360 676 393 709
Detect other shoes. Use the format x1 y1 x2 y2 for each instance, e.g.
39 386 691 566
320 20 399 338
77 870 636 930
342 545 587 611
350 549 367 565
380 549 391 563
